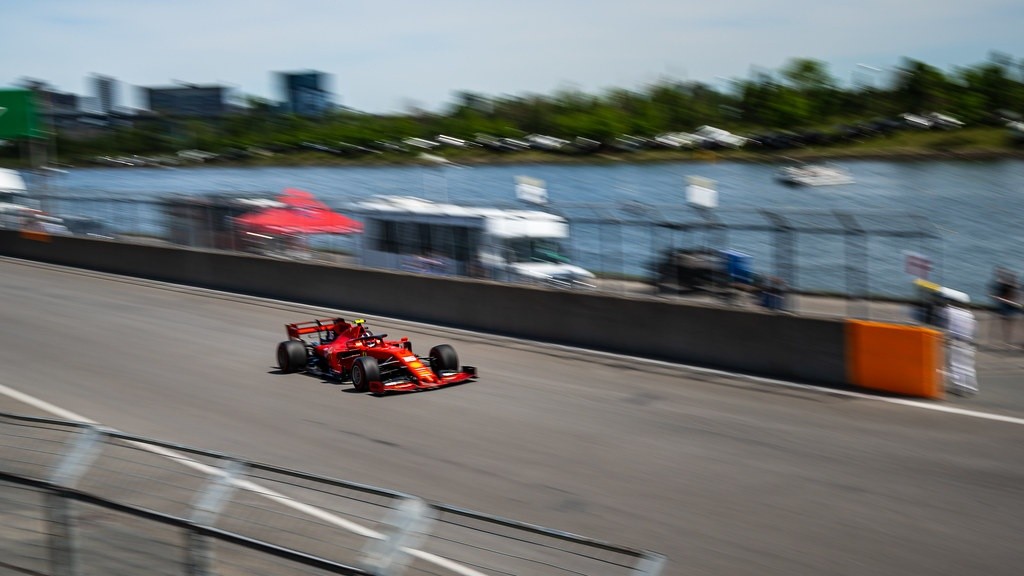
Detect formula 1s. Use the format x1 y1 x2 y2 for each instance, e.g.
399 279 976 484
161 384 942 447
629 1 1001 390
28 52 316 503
276 317 479 398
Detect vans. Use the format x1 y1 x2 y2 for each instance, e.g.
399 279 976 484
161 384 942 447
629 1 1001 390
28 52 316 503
356 195 598 296
1 164 72 237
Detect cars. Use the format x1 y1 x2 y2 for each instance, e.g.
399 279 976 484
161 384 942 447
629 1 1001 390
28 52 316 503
164 183 365 270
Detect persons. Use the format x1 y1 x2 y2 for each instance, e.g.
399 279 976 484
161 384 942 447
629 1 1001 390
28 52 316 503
322 317 345 344
986 264 1024 351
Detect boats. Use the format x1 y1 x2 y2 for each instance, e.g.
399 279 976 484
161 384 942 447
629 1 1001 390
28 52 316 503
773 164 855 190
